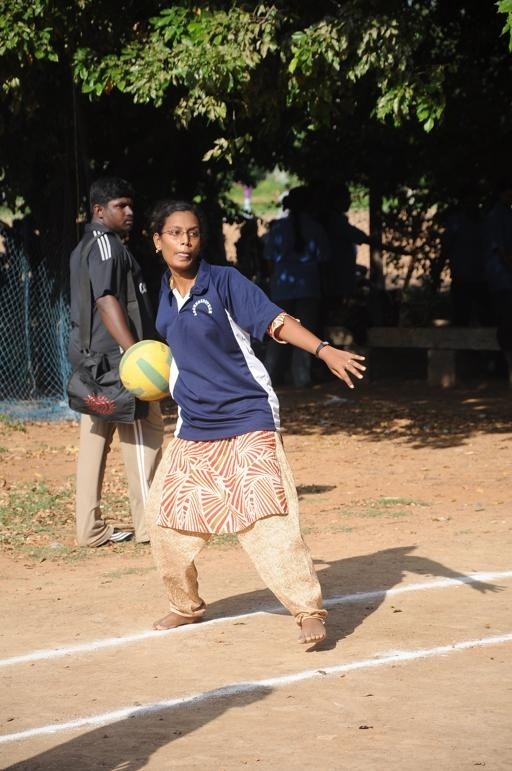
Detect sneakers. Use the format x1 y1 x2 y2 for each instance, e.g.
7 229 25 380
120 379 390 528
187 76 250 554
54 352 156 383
108 527 133 544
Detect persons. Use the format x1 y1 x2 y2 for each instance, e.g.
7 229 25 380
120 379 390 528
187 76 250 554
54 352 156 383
432 179 512 385
67 181 164 549
236 186 414 390
144 202 366 645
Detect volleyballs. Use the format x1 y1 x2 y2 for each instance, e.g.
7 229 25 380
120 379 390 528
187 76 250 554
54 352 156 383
120 340 170 402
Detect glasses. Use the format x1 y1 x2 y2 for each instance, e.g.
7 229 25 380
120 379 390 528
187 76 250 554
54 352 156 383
157 230 200 238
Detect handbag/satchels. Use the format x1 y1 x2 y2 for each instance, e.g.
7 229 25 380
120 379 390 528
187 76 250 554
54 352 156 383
66 352 151 422
